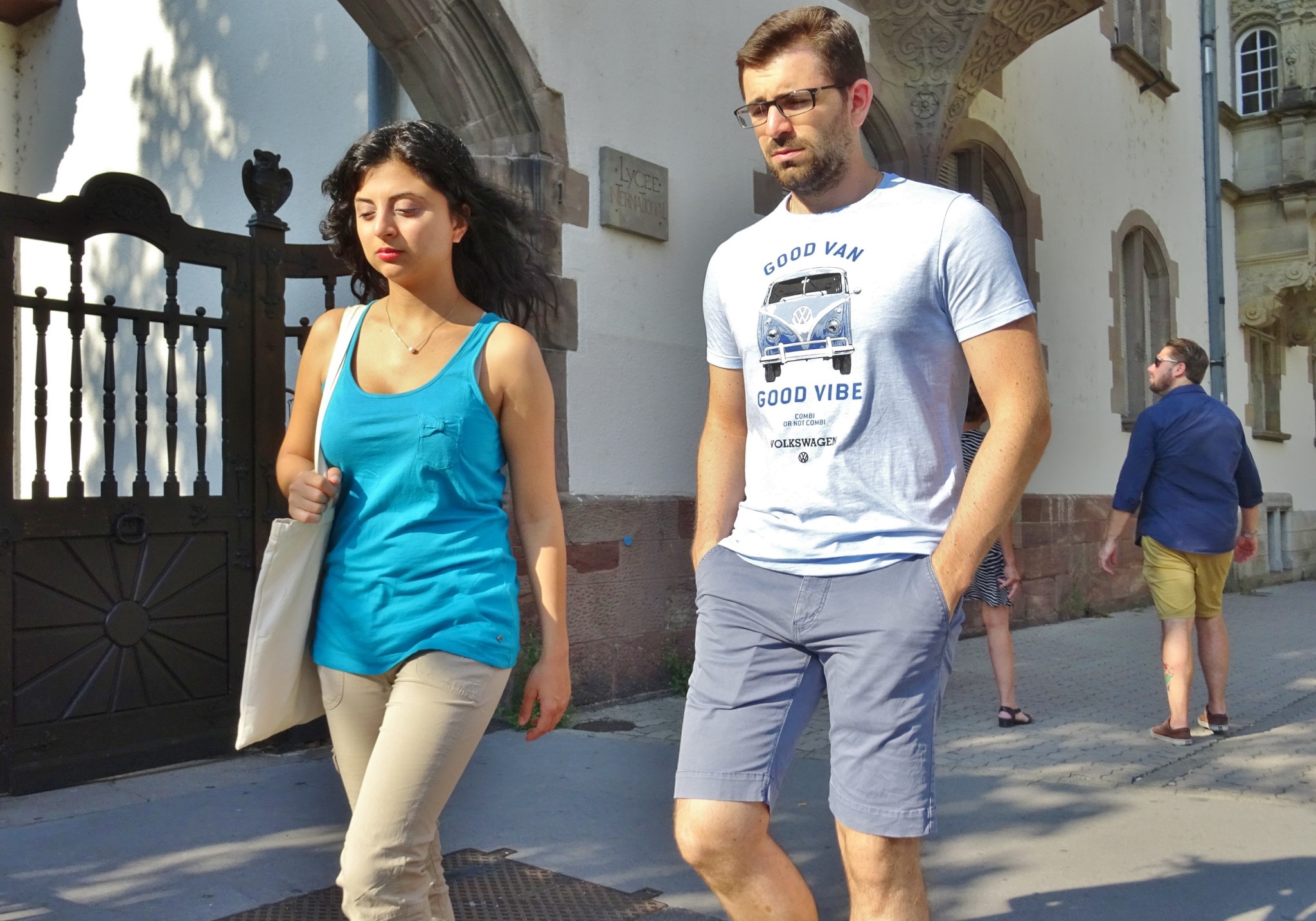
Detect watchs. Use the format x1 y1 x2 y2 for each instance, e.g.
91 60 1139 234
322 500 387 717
1239 527 1259 538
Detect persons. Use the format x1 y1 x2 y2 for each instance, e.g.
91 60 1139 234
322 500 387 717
1095 337 1264 748
669 5 1054 921
958 370 1035 727
276 119 573 921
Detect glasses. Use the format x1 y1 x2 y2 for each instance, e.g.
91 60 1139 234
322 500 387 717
1154 356 1177 368
733 82 844 129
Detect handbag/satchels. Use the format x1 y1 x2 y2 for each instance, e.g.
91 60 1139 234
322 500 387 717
235 507 326 749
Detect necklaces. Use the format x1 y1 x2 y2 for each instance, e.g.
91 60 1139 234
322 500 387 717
385 292 460 353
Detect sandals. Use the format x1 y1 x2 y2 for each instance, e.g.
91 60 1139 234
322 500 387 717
998 705 1032 727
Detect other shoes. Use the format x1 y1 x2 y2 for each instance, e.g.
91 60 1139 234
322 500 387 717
1197 704 1229 730
1150 716 1193 745
